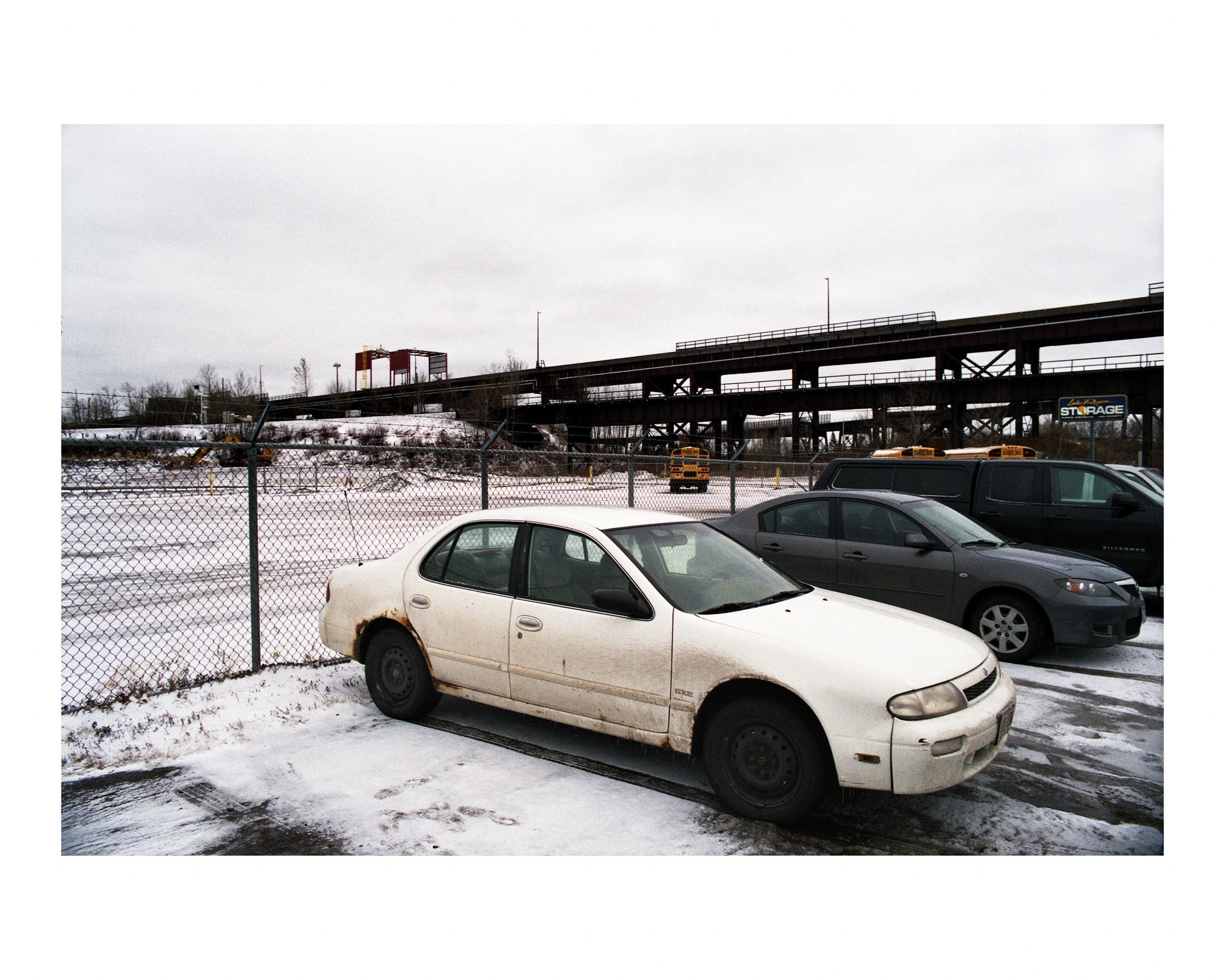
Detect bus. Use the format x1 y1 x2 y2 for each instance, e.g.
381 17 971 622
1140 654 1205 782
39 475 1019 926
864 443 949 459
942 445 1037 460
669 445 710 493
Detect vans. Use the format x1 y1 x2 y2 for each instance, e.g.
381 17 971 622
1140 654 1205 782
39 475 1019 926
814 459 1164 589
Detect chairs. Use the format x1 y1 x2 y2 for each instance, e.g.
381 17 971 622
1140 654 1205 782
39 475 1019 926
763 506 902 547
434 544 631 608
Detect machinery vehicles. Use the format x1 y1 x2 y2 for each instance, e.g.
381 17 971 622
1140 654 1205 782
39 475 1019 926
169 430 275 469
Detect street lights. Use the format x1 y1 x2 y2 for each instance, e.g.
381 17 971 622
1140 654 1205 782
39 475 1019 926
825 278 830 332
333 363 341 394
536 311 542 368
260 364 263 401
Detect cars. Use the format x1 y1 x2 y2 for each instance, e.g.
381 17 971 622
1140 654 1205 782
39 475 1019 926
319 505 1016 822
687 489 1146 663
